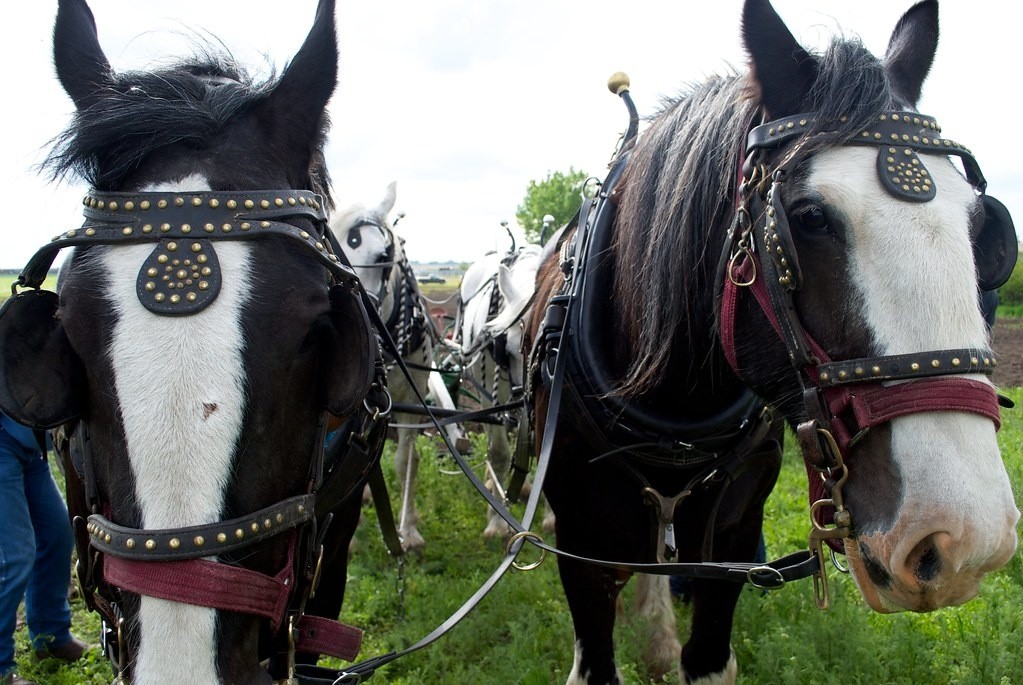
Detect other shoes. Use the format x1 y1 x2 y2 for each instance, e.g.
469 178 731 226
0 671 37 685
31 635 91 665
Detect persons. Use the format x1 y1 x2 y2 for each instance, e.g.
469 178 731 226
1 408 91 685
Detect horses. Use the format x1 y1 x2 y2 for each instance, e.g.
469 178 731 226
457 0 1021 685
30 0 432 685
